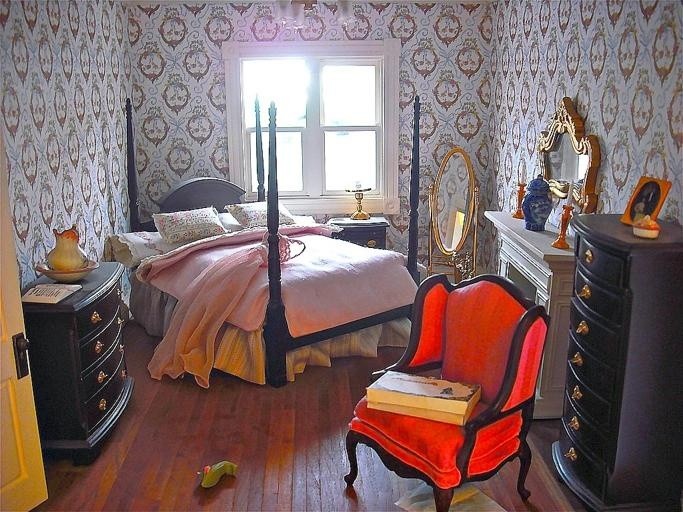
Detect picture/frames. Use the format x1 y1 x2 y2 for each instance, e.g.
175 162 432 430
620 175 672 227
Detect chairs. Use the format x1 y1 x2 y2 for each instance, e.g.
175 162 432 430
344 274 550 510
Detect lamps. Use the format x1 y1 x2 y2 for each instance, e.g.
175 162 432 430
344 183 371 220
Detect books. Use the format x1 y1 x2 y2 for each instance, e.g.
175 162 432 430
21 283 82 305
365 369 481 427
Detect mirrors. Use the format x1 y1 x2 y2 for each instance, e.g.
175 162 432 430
427 149 479 281
537 97 600 230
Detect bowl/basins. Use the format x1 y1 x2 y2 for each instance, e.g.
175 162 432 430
36 261 99 283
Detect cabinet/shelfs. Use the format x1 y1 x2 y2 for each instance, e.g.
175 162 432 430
552 214 683 511
20 263 135 467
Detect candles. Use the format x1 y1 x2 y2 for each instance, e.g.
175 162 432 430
520 158 527 183
567 179 574 207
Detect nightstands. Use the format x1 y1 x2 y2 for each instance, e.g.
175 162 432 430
329 215 388 251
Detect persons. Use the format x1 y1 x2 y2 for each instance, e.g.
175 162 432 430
634 197 645 220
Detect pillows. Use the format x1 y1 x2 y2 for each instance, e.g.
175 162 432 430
150 208 229 243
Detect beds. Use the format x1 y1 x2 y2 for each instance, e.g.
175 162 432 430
102 93 428 389
225 200 295 229
